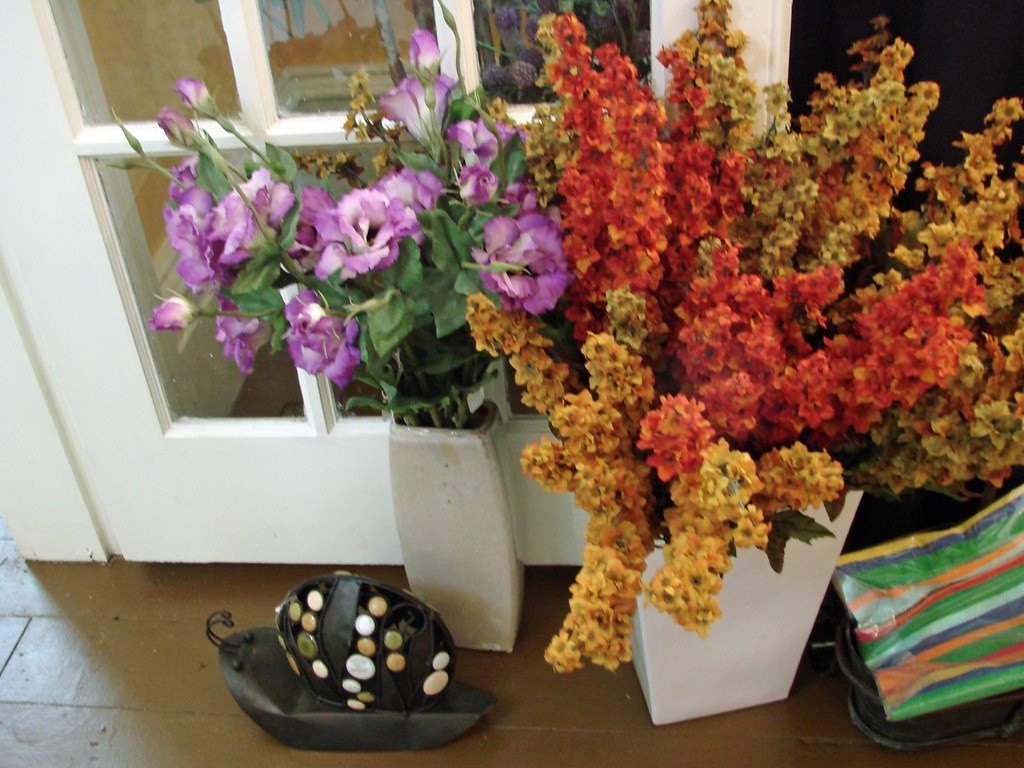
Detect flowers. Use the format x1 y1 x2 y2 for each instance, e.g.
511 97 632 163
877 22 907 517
446 0 1024 675
109 27 565 423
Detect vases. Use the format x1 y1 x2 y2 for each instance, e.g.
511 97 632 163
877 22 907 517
624 489 864 728
387 423 525 656
